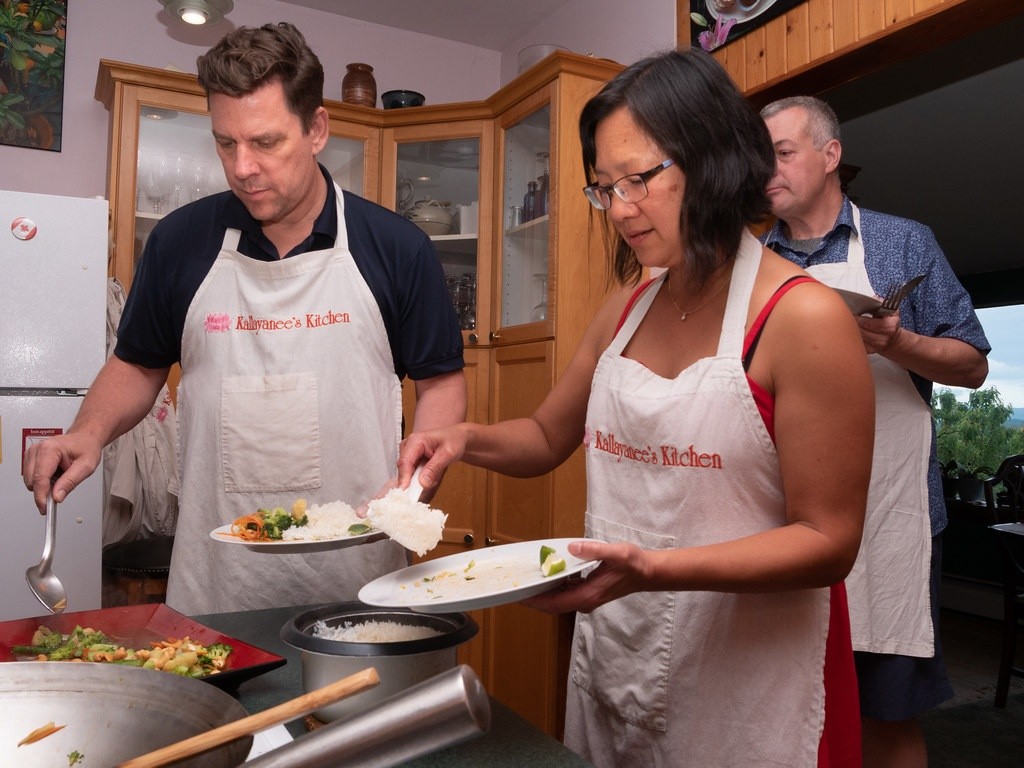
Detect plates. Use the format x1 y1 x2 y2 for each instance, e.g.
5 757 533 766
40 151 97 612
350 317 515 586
0 602 287 684
357 538 610 612
705 0 778 24
831 287 883 316
209 523 387 554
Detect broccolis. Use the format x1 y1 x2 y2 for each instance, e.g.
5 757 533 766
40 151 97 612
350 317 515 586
9 624 233 679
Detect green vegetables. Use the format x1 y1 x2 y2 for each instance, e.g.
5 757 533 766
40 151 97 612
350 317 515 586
247 508 309 539
348 523 372 535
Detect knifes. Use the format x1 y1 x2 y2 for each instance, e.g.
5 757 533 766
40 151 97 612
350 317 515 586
892 275 926 302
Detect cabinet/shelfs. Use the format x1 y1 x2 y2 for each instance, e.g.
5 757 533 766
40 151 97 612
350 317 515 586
94 49 624 736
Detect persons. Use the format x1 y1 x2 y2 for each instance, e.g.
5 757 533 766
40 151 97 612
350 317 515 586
752 97 991 768
399 52 874 768
23 24 468 616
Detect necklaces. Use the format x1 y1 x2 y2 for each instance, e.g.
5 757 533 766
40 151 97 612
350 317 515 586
664 275 728 321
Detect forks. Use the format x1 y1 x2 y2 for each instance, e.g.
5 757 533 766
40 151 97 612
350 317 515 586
878 283 905 319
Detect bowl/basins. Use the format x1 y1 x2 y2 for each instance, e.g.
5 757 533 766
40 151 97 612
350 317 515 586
518 44 572 77
381 90 425 110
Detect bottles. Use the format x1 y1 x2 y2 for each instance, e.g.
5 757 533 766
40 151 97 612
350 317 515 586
342 62 377 107
523 155 550 223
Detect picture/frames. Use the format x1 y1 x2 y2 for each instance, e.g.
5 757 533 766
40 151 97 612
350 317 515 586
0 0 68 152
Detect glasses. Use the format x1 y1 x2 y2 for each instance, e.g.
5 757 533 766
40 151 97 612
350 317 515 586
583 158 676 210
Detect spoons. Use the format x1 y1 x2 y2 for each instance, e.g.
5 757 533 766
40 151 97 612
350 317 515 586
25 489 67 615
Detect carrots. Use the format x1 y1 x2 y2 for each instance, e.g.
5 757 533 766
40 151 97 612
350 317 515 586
214 511 272 540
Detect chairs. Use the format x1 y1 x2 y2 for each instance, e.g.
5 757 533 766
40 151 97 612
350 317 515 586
984 455 1024 709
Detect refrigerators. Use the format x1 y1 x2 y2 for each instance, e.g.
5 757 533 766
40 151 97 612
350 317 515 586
2 190 111 616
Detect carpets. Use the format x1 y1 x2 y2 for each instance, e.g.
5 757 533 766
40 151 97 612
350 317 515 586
913 692 1024 768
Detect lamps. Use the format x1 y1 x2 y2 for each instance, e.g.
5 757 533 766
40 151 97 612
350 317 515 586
157 0 234 26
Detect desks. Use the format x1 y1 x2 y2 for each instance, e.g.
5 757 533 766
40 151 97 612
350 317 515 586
986 521 1024 536
189 602 586 768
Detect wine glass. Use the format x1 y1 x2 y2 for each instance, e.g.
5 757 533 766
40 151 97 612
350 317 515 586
530 273 547 323
135 148 213 215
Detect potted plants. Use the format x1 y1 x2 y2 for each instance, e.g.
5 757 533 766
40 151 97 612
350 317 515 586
955 460 995 501
938 462 962 499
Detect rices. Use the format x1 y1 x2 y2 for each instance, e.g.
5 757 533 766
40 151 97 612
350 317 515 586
280 500 369 541
312 619 446 643
366 487 449 557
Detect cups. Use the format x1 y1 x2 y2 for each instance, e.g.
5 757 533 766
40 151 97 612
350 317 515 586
736 0 760 11
509 205 522 228
444 274 476 330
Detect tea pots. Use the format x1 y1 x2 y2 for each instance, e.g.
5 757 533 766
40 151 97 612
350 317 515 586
398 179 460 236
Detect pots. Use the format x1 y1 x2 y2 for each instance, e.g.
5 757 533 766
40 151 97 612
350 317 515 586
0 660 491 768
281 602 480 723
